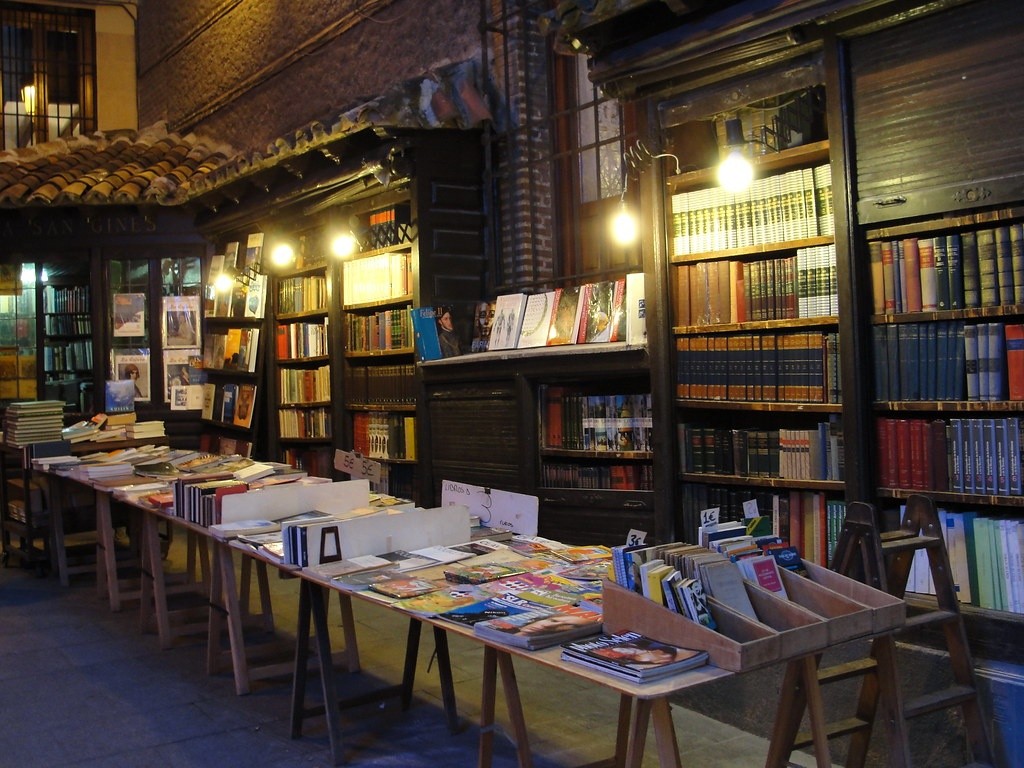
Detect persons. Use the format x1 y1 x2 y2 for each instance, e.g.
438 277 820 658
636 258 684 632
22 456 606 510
437 311 462 358
506 615 593 638
472 301 496 353
125 364 142 398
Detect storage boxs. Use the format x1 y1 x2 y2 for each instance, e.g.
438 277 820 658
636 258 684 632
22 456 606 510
602 558 906 672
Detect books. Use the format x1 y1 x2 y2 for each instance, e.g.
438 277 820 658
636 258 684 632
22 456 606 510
302 490 845 684
667 162 845 479
97 209 414 562
2 287 97 488
868 222 1023 613
413 273 654 490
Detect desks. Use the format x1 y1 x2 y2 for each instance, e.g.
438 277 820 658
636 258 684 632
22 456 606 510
0 435 903 768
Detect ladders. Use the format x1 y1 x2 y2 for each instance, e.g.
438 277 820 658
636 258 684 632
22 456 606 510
765 493 1000 768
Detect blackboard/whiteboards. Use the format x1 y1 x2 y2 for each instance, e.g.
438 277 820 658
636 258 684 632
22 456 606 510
333 449 381 484
442 479 539 537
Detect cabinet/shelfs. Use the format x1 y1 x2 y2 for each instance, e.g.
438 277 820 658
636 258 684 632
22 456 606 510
416 356 654 545
44 275 91 375
587 0 1024 665
199 183 418 508
